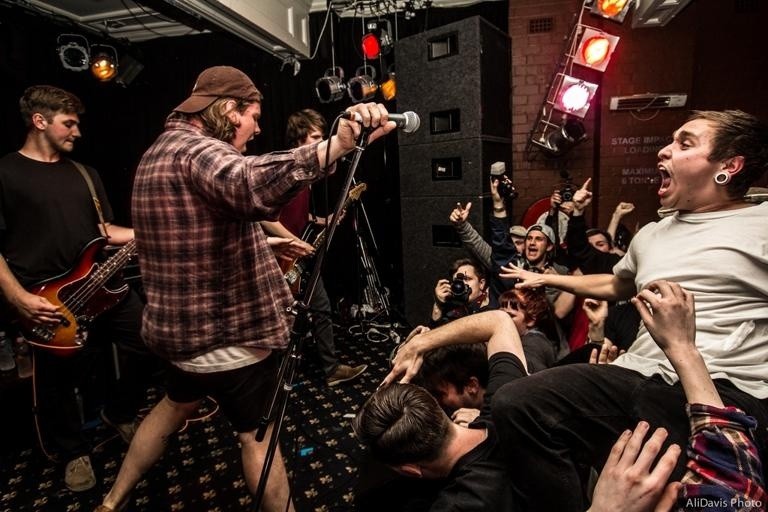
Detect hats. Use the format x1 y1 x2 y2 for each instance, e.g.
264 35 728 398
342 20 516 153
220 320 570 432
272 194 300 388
509 225 526 237
526 223 555 245
171 65 263 114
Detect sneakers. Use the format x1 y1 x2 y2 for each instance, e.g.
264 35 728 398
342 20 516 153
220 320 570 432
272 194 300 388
100 408 141 445
64 456 97 493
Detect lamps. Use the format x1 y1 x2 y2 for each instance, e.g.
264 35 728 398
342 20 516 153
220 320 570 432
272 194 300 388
543 0 684 155
315 0 399 104
55 31 143 90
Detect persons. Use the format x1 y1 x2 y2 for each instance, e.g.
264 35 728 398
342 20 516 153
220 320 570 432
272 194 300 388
1 65 768 511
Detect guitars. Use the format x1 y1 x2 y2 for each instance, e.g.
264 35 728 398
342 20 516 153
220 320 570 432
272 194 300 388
274 182 367 301
12 236 137 352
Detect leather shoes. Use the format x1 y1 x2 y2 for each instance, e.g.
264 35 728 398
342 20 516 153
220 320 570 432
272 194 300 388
326 364 368 387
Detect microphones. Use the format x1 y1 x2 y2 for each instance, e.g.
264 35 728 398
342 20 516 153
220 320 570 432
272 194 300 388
345 100 420 134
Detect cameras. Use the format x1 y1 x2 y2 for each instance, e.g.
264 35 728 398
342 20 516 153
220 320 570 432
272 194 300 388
561 188 573 202
490 161 519 201
449 273 471 303
